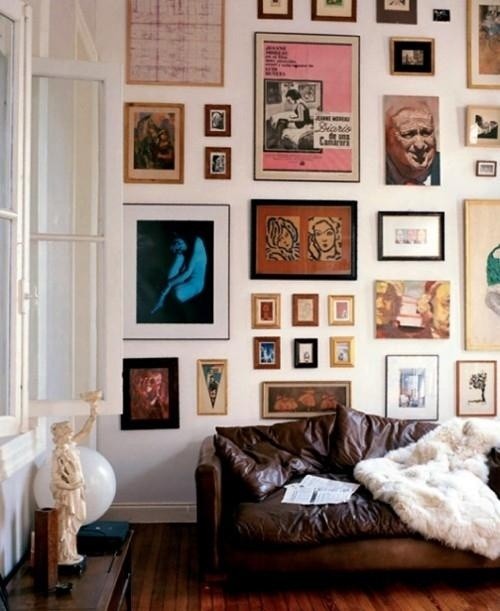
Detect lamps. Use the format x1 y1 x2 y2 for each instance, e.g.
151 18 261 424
33 446 130 555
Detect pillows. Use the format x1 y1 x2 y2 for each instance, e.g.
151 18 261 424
214 413 336 502
320 403 441 472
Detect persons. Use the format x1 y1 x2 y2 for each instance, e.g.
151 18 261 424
374 280 412 338
297 389 317 412
208 376 218 409
265 216 300 262
130 372 157 420
307 216 342 262
413 281 450 338
281 89 313 150
385 99 440 186
151 372 167 419
50 405 100 566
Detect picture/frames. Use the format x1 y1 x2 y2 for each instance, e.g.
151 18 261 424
456 360 498 417
249 199 358 282
373 279 451 340
385 355 438 420
292 293 319 326
126 0 225 87
262 380 352 419
467 0 500 90
122 357 180 431
254 32 360 182
122 102 185 184
253 336 281 369
311 0 356 22
330 336 356 368
464 199 500 352
476 161 497 177
379 91 444 189
389 37 436 77
294 338 318 368
258 0 293 19
378 211 445 261
376 0 418 24
251 293 281 329
204 104 231 138
204 147 232 180
196 358 228 416
465 105 500 148
123 204 230 339
328 295 355 326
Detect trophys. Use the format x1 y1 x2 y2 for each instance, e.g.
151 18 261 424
80 389 103 418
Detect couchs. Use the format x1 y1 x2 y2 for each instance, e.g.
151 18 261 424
194 434 500 592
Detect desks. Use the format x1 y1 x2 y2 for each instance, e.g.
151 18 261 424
0 525 136 611
